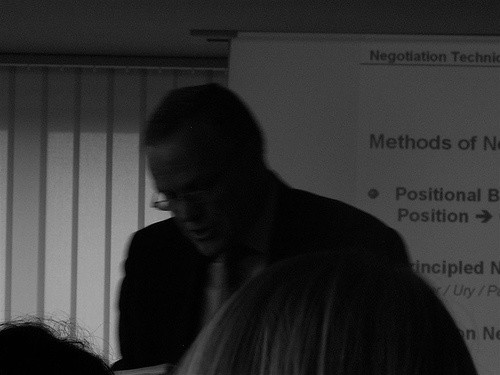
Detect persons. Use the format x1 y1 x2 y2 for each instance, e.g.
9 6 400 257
170 242 421 375
117 83 480 375
0 314 117 375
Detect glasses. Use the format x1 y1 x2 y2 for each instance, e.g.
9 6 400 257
150 171 232 212
